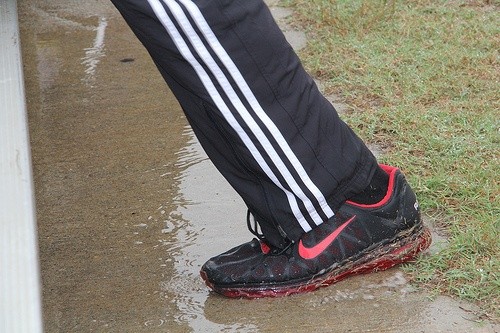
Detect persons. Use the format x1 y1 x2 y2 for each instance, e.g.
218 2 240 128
109 0 434 301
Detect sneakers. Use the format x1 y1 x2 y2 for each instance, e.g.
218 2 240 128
199 166 432 297
